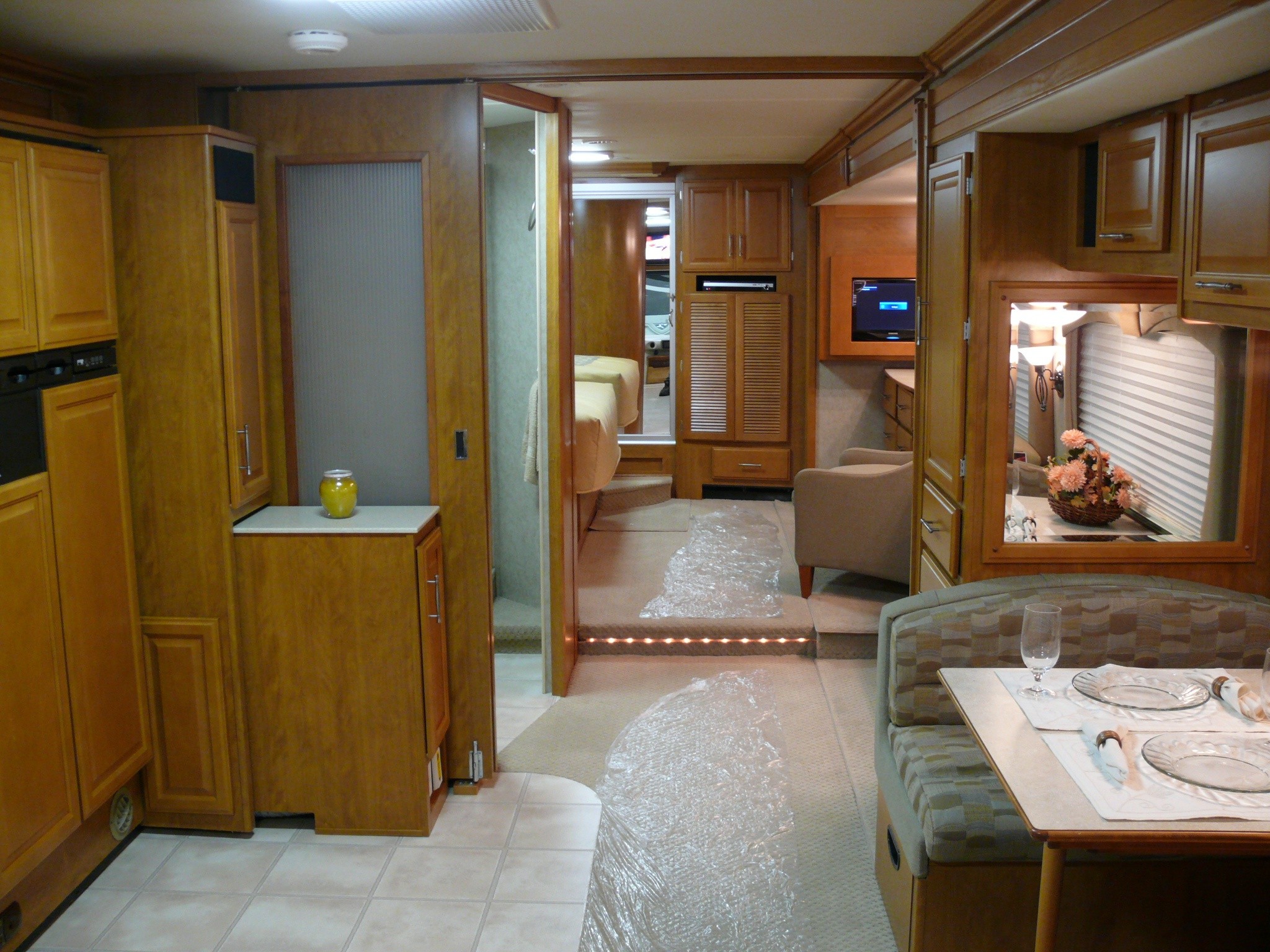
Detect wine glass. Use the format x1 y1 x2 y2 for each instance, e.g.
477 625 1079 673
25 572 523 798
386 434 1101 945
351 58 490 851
1019 604 1063 698
1259 649 1270 752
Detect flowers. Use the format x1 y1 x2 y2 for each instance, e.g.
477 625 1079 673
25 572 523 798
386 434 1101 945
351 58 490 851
1035 424 1137 512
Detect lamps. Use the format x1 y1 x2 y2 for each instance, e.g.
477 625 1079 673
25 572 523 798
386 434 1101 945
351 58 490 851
1020 344 1064 415
568 146 614 165
1007 342 1021 405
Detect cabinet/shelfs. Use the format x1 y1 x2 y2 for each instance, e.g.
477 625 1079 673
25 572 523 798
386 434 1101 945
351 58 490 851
916 142 972 602
0 75 1268 912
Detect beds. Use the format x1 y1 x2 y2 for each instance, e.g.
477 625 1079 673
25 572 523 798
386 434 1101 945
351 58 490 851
572 352 643 490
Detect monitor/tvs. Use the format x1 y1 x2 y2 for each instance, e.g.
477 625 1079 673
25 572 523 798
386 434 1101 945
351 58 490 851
851 278 918 342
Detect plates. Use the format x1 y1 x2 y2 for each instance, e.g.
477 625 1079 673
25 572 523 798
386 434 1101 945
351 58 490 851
1071 667 1211 711
1141 732 1270 792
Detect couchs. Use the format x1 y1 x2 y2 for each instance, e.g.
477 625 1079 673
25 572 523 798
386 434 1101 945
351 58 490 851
872 574 1270 952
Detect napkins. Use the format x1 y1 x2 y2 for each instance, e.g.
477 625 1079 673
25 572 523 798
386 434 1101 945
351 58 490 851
994 667 1269 733
1037 725 1270 823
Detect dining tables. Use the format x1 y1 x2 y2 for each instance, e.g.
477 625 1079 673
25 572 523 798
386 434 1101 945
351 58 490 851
936 665 1270 952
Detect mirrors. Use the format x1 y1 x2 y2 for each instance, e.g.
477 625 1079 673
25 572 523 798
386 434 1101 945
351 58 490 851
983 276 1270 570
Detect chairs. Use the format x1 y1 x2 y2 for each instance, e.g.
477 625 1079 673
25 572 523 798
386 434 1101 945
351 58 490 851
789 445 918 602
